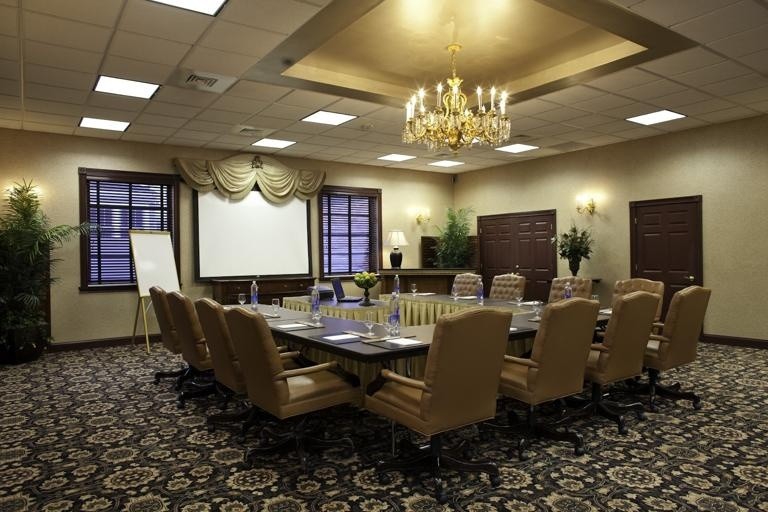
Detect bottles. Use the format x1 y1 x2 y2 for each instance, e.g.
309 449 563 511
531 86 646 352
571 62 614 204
476 278 483 305
311 285 320 320
452 284 459 301
387 292 402 337
564 281 572 299
250 281 258 310
393 274 400 296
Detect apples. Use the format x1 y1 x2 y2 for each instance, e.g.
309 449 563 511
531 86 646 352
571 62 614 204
354 271 377 283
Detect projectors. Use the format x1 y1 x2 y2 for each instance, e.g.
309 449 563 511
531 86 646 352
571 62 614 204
308 285 333 299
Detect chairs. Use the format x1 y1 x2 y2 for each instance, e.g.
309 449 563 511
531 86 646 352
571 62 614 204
633 285 712 413
548 277 592 302
167 291 213 389
365 305 512 489
223 306 359 453
489 274 525 300
613 278 664 321
451 273 483 298
178 297 300 432
149 286 187 385
573 291 662 434
498 297 601 461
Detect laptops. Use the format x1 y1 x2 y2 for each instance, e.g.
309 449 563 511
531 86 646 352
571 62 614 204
331 278 363 302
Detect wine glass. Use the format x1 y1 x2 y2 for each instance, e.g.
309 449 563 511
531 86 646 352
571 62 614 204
533 298 542 319
271 298 280 317
363 312 376 336
514 288 524 308
238 294 246 308
313 311 323 325
410 284 417 296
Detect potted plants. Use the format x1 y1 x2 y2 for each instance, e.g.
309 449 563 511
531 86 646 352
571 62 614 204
549 216 598 276
0 177 103 366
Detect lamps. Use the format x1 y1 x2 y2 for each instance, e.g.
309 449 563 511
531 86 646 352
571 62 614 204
386 230 410 268
396 18 512 157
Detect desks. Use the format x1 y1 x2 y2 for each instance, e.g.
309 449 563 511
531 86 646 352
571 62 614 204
225 292 611 377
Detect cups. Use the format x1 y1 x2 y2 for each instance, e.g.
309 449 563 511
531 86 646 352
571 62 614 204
383 320 400 338
592 295 599 301
